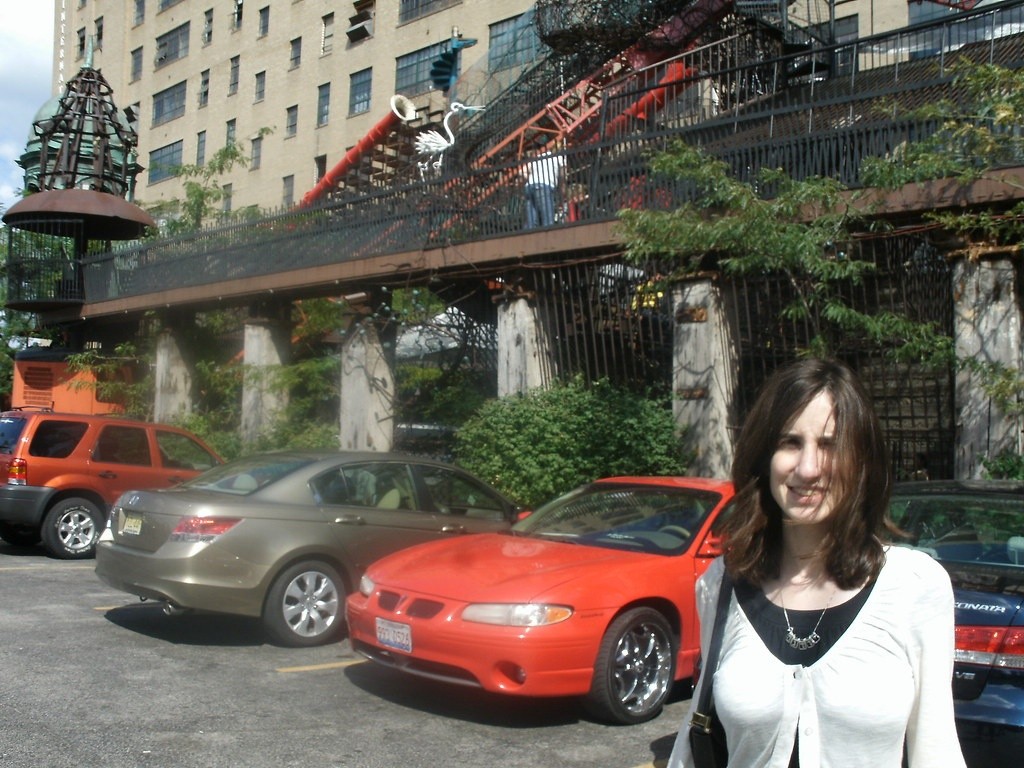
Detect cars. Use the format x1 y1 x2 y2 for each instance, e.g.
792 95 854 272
94 449 538 649
877 479 1024 730
345 475 736 725
0 406 225 560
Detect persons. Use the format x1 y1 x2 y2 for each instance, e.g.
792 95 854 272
665 358 970 768
517 145 566 229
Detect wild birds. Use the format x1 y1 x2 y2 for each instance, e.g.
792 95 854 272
413 101 486 183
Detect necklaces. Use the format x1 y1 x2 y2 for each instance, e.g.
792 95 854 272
778 578 836 650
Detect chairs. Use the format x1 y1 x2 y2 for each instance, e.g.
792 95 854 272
330 464 414 511
1006 535 1024 566
429 494 453 514
654 496 705 534
98 437 122 463
